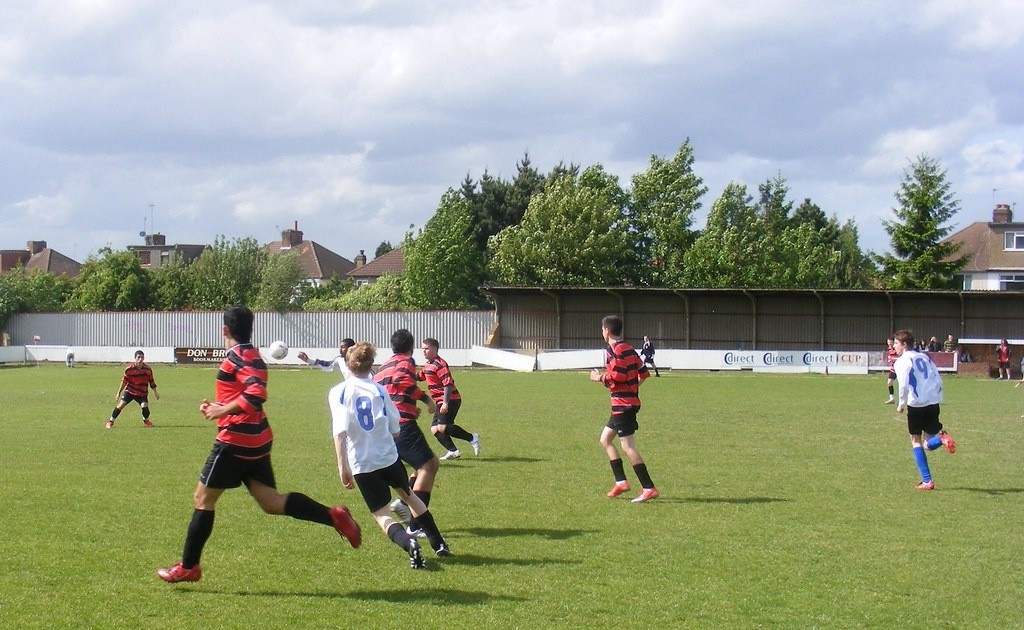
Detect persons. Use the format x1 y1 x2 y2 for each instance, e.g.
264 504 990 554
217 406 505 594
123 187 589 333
591 316 660 502
943 334 956 351
415 338 482 460
885 337 901 405
892 330 957 489
373 327 440 538
638 336 660 377
913 339 919 351
66 345 75 368
995 338 1011 380
1014 378 1024 388
918 340 929 352
328 341 452 570
105 350 160 428
155 303 364 583
928 335 941 352
297 338 376 380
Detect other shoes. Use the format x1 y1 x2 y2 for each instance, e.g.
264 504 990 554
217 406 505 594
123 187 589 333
997 377 1003 380
656 373 660 377
886 399 895 403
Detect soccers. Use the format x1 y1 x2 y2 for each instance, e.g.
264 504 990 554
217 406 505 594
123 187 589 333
269 341 288 360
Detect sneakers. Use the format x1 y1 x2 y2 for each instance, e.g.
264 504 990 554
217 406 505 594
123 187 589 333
390 499 411 523
435 543 450 556
406 525 427 538
939 430 956 453
440 449 461 460
158 561 202 582
330 505 362 548
631 487 659 503
914 480 934 490
408 538 426 569
144 419 153 426
606 481 631 497
470 432 480 456
106 421 114 428
